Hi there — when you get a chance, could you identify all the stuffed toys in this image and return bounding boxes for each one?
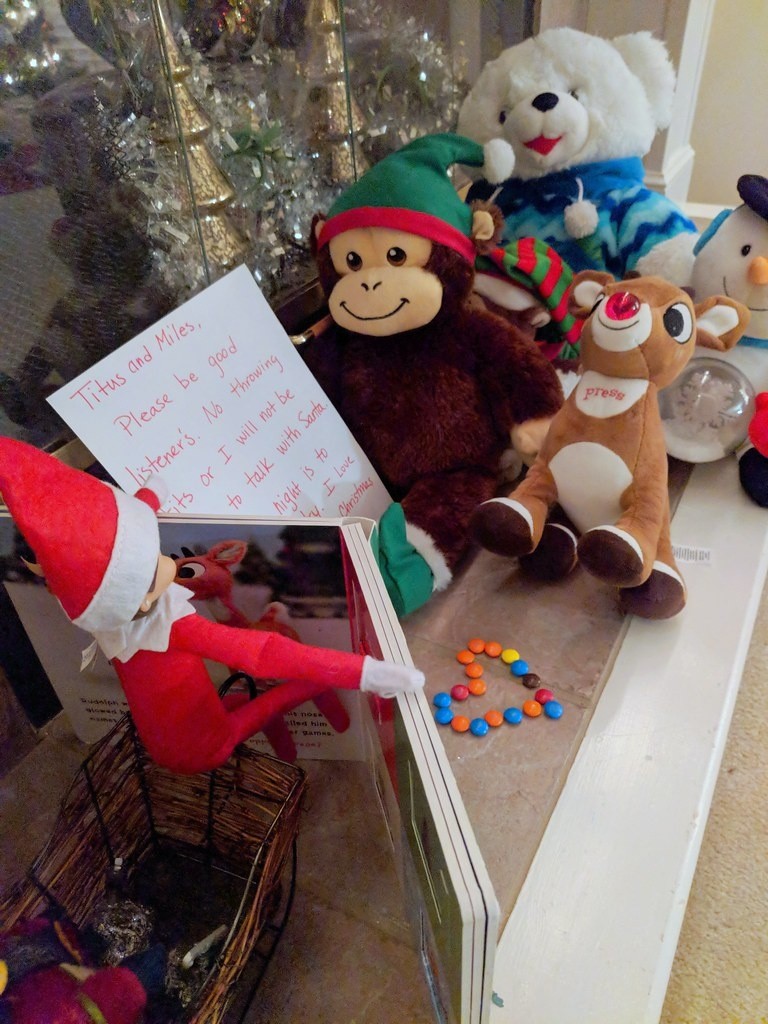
[691,174,768,510]
[296,131,566,622]
[470,270,751,623]
[455,27,700,286]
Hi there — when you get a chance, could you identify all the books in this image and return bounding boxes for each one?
[0,505,501,1024]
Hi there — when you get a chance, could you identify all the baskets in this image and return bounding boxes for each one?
[0,711,308,1024]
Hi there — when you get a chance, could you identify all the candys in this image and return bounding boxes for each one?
[433,639,566,736]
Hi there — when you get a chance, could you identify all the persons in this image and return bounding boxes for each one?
[0,435,424,778]
